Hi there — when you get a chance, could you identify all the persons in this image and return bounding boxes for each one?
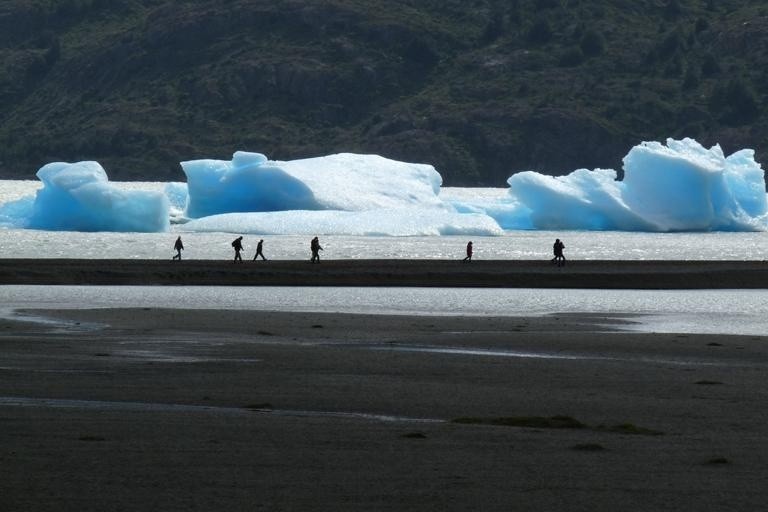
[463,242,472,263]
[232,237,244,260]
[552,239,565,266]
[254,240,267,260]
[311,237,323,264]
[173,237,184,261]
